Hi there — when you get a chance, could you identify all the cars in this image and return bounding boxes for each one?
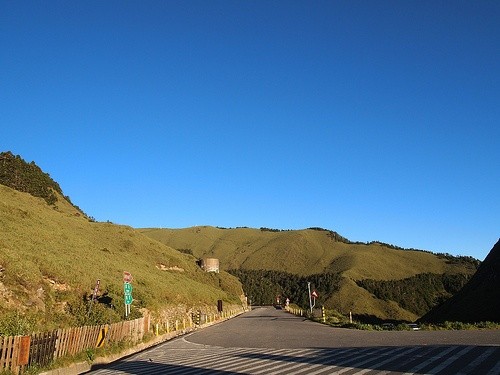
[381,323,395,331]
[406,324,421,331]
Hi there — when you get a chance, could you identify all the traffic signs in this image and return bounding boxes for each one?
[124,272,133,304]
